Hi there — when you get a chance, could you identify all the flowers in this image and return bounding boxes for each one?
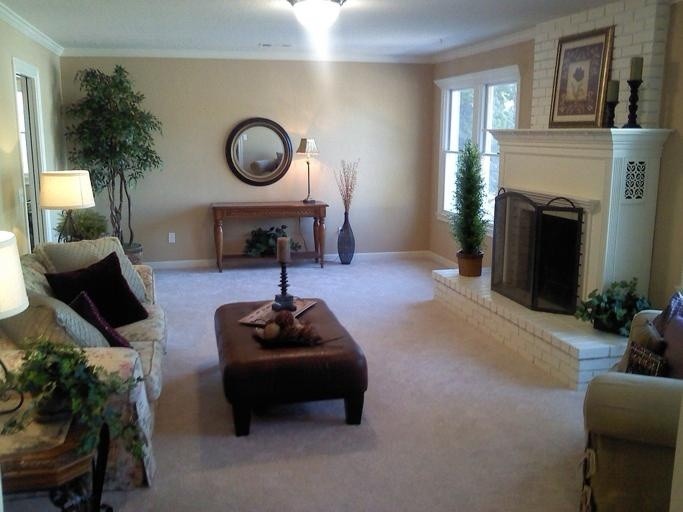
[332,158,361,213]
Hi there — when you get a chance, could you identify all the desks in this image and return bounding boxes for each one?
[211,201,329,273]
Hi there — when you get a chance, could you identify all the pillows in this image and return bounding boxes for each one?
[44,236,147,306]
[625,341,668,376]
[653,292,683,337]
[65,291,134,347]
[44,250,148,328]
[4,289,110,348]
[633,319,665,352]
[661,312,683,380]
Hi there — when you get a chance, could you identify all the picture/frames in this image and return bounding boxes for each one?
[547,26,615,129]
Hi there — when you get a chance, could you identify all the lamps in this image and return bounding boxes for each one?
[0,230,31,321]
[287,1,347,26]
[37,170,94,239]
[296,137,319,202]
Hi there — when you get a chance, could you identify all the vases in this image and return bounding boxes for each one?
[338,213,355,265]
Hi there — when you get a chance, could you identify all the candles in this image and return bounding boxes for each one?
[629,56,643,81]
[607,80,619,101]
[277,236,291,263]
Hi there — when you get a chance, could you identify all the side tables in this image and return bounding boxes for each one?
[1,388,101,504]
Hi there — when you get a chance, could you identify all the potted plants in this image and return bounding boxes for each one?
[451,137,488,277]
[574,277,653,336]
[242,224,301,256]
[59,61,163,265]
[3,342,146,470]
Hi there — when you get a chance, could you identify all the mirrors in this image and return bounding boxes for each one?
[226,117,294,186]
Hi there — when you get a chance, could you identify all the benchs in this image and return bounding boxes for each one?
[214,298,368,437]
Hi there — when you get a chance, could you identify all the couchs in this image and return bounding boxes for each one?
[1,252,167,488]
[582,311,683,512]
[250,152,283,176]
